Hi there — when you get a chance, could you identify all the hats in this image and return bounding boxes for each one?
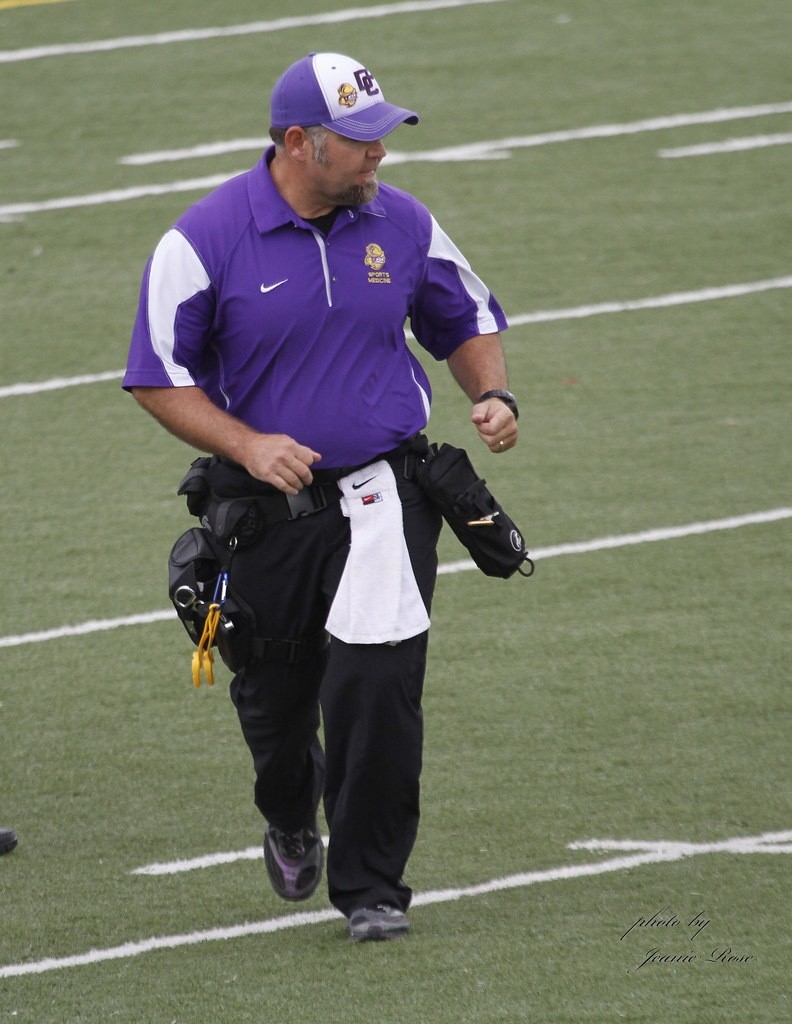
[269,52,418,140]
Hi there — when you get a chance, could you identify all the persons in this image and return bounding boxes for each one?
[121,53,519,941]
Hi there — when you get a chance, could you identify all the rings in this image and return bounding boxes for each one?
[499,440,504,451]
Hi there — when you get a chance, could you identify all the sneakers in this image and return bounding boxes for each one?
[264,826,323,901]
[349,904,407,941]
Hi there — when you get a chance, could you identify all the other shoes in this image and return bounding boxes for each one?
[2,826,17,853]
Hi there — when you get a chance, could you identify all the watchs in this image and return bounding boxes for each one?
[477,389,519,422]
